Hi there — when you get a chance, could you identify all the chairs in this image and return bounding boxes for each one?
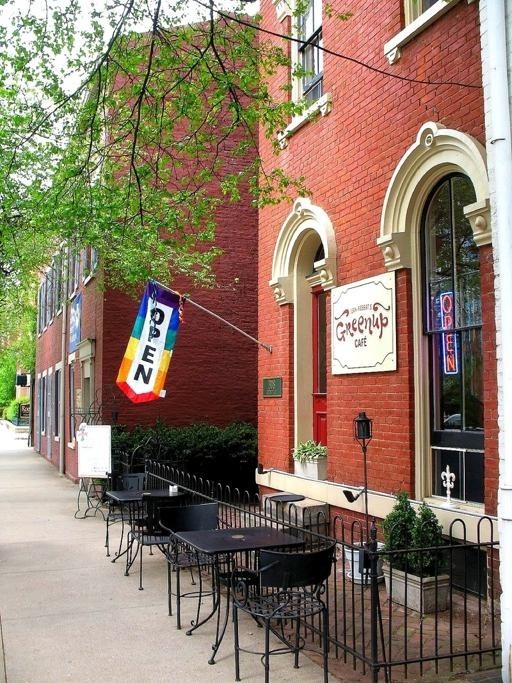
[94,467,153,557]
[157,502,236,631]
[124,493,197,590]
[230,540,334,682]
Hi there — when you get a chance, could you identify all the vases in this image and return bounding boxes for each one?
[344,540,387,579]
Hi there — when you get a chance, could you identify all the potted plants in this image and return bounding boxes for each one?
[379,482,457,616]
[289,439,329,481]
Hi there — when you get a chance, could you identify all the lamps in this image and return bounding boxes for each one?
[258,462,271,474]
[343,487,365,504]
[352,411,374,542]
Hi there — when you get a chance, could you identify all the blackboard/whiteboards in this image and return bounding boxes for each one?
[78,425,112,478]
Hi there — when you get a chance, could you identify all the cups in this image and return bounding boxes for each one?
[169,484,178,492]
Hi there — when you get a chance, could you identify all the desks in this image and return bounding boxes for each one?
[106,488,186,575]
[173,528,307,664]
